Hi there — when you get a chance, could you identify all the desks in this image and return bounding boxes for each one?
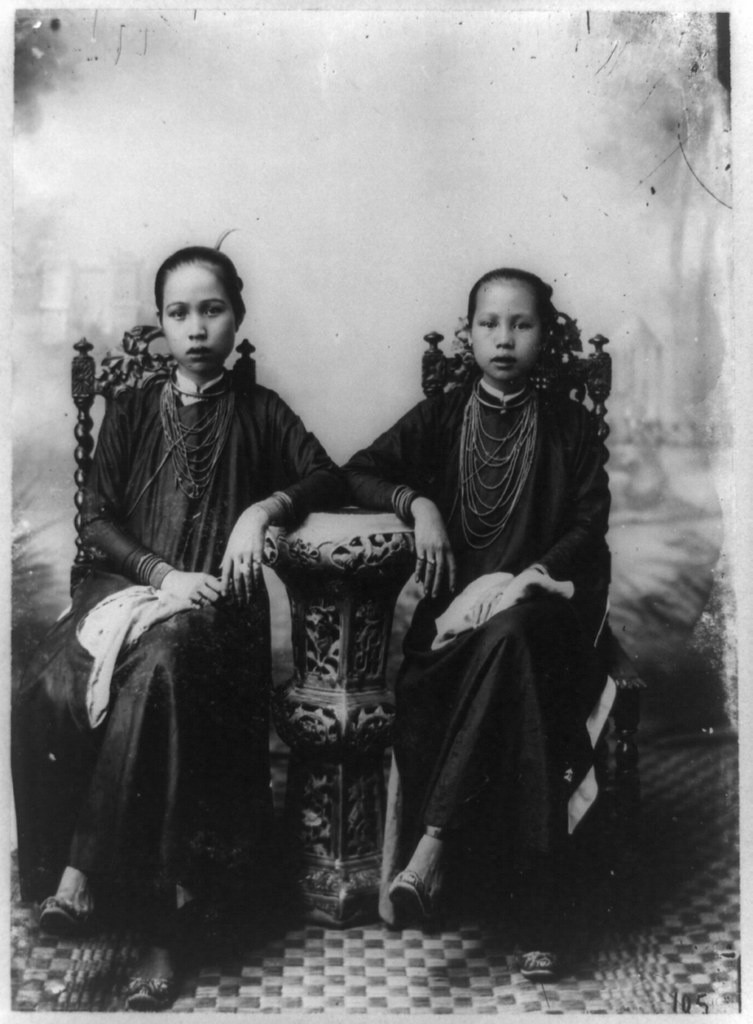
[262,505,418,930]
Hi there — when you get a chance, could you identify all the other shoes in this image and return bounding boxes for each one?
[127,966,177,1006]
[41,895,98,934]
[515,950,563,978]
[388,868,444,919]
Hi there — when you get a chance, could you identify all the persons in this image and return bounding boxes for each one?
[39,247,344,1014]
[338,267,618,975]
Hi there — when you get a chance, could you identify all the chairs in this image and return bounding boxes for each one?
[419,329,650,923]
[69,327,275,911]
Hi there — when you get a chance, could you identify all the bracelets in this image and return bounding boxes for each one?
[391,483,415,523]
[139,554,174,589]
[255,491,294,521]
[533,566,544,574]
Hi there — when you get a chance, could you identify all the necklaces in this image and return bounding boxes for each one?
[458,374,539,549]
[160,362,238,500]
